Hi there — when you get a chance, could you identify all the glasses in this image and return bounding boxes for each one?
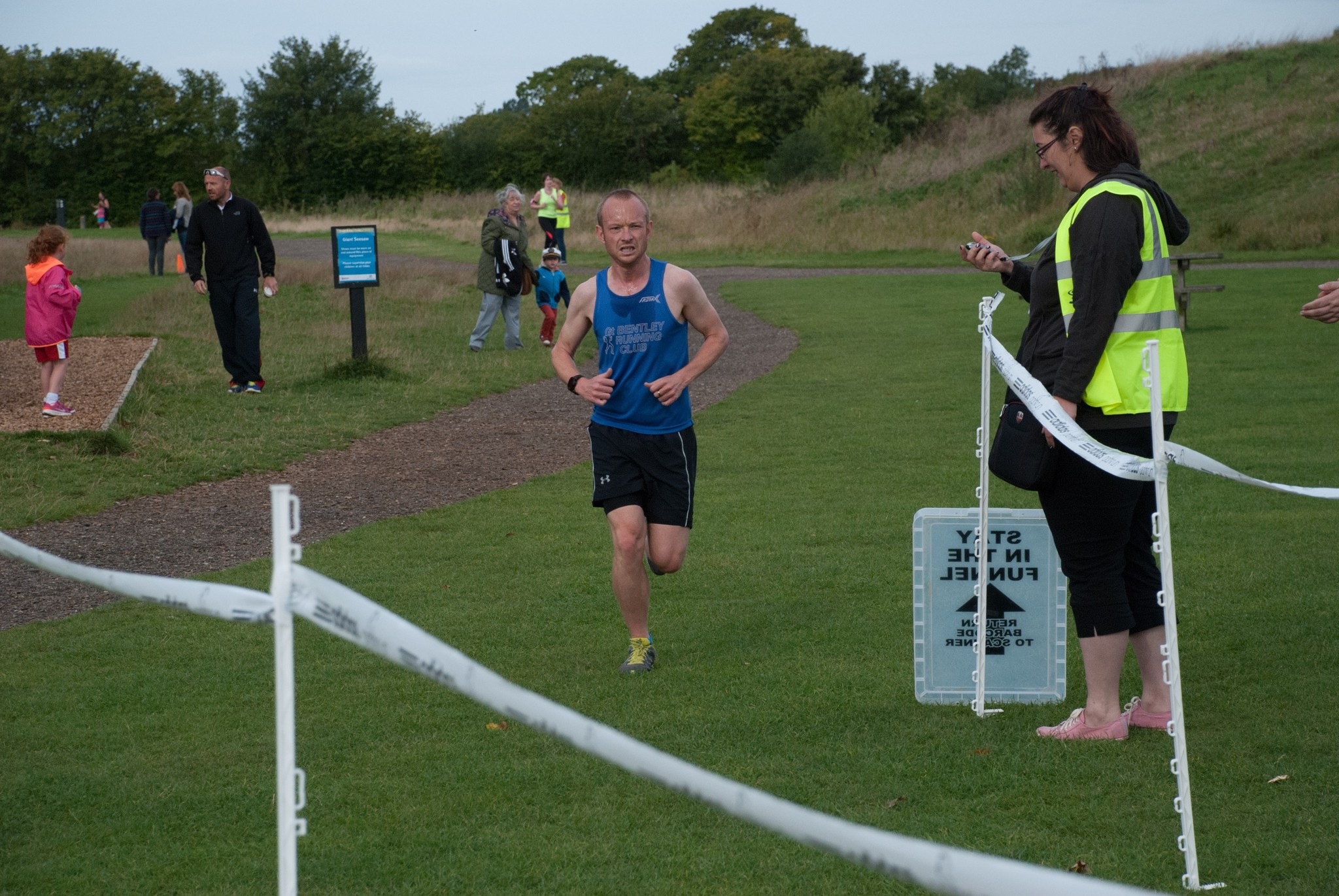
[204,169,228,180]
[1034,132,1067,159]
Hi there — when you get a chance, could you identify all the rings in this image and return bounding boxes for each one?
[657,390,662,397]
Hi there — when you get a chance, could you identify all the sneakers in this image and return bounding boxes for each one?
[644,536,665,575]
[619,634,656,676]
[43,407,76,418]
[42,402,72,416]
[1121,696,1173,731]
[244,381,261,393]
[1037,708,1129,741]
[227,381,246,393]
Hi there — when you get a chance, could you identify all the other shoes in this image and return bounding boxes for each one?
[540,334,550,345]
[558,260,567,266]
[470,346,480,352]
[544,342,556,347]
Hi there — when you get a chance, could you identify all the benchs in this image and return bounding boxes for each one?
[1170,253,1227,331]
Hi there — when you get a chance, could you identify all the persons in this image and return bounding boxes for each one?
[535,247,571,349]
[139,187,171,276]
[171,181,194,272]
[92,192,111,229]
[25,225,81,418]
[469,184,534,352]
[185,167,278,392]
[958,86,1192,741]
[530,173,563,268]
[1300,278,1339,324]
[550,189,729,677]
[551,177,570,266]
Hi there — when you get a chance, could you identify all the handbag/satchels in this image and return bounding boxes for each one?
[989,350,1059,490]
[521,267,532,295]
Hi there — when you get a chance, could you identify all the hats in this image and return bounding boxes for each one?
[542,247,561,263]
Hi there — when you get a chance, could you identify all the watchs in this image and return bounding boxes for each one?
[567,374,585,395]
